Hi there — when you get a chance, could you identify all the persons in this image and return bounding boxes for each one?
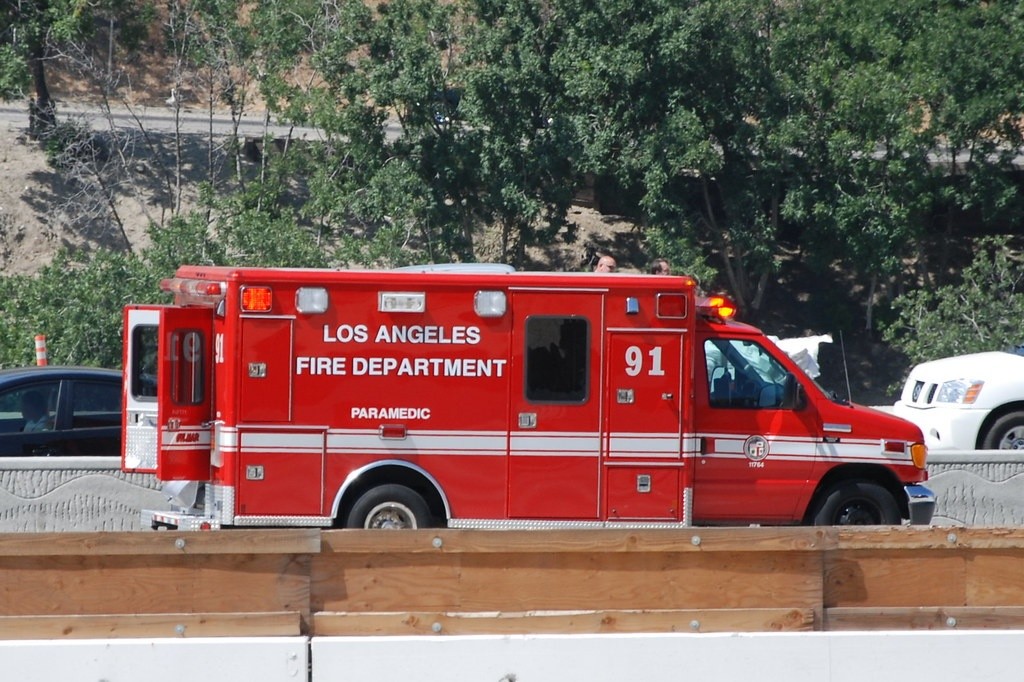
[19,389,52,434]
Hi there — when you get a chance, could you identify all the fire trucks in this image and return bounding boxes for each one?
[119,263,942,534]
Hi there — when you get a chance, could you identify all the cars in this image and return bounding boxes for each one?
[0,366,156,460]
[891,339,1024,450]
